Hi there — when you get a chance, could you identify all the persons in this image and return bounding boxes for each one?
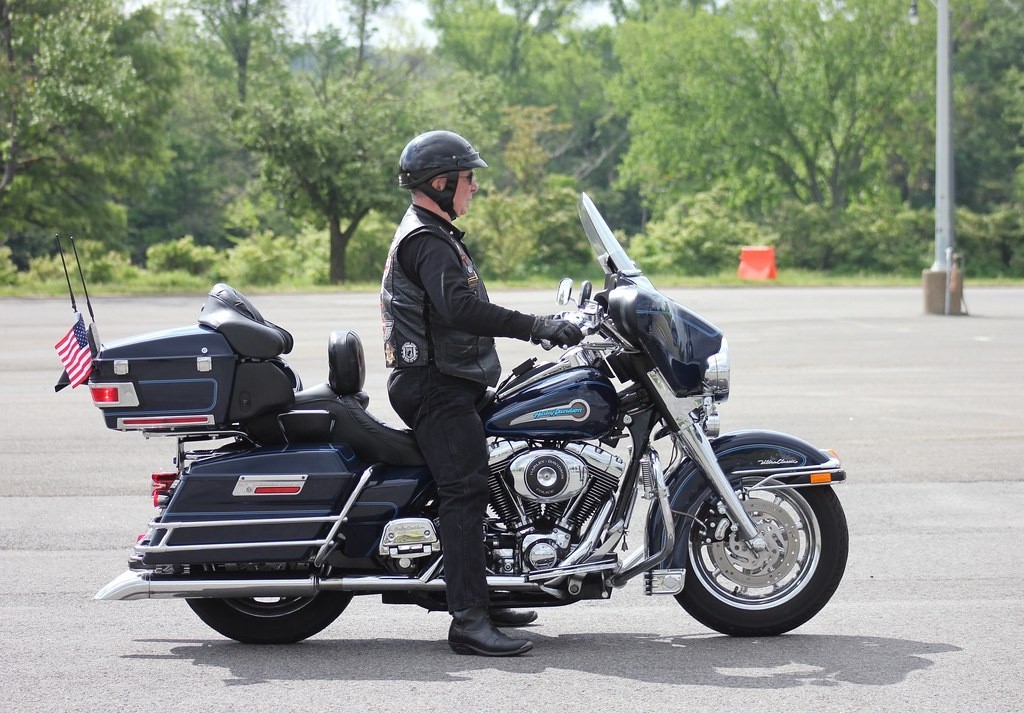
[379,130,585,658]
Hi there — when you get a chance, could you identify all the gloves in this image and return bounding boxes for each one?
[531,316,582,347]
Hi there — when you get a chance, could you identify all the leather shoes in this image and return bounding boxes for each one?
[487,605,537,626]
[448,606,535,656]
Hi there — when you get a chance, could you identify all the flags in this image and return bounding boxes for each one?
[55,316,93,389]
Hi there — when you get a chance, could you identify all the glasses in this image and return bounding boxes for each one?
[433,171,473,186]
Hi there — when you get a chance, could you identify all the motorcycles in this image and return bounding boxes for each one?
[85,189,852,645]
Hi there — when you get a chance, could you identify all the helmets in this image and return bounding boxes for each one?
[398,131,487,190]
